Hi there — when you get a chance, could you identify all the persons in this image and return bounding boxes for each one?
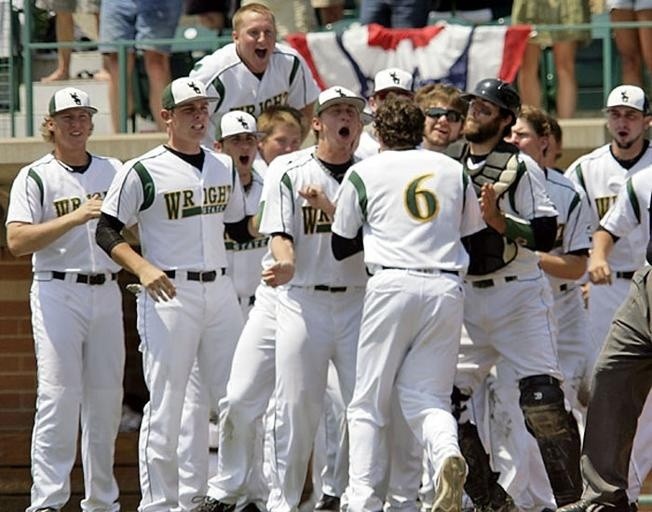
[257,108,304,173]
[258,86,371,512]
[190,121,365,512]
[179,110,288,512]
[355,69,652,512]
[511,1,592,116]
[5,87,140,512]
[31,1,230,134]
[606,0,652,91]
[189,3,320,149]
[94,75,256,512]
[361,1,513,29]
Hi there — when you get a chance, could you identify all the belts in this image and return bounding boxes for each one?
[53,271,116,285]
[164,268,225,282]
[315,284,347,292]
[616,271,634,279]
[463,276,516,287]
[238,296,255,304]
[365,266,459,276]
[560,284,567,290]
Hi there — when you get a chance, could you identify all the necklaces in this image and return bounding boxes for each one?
[314,153,345,179]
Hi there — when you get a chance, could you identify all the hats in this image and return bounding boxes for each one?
[606,84,650,113]
[315,85,365,117]
[460,79,522,125]
[375,68,414,97]
[50,88,97,117]
[159,77,219,110]
[216,109,268,144]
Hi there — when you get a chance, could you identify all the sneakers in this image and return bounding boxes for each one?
[472,486,518,512]
[431,455,467,511]
[126,112,159,133]
[555,497,631,512]
[313,493,340,511]
[189,495,235,511]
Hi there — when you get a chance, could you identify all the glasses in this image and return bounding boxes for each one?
[425,108,466,123]
